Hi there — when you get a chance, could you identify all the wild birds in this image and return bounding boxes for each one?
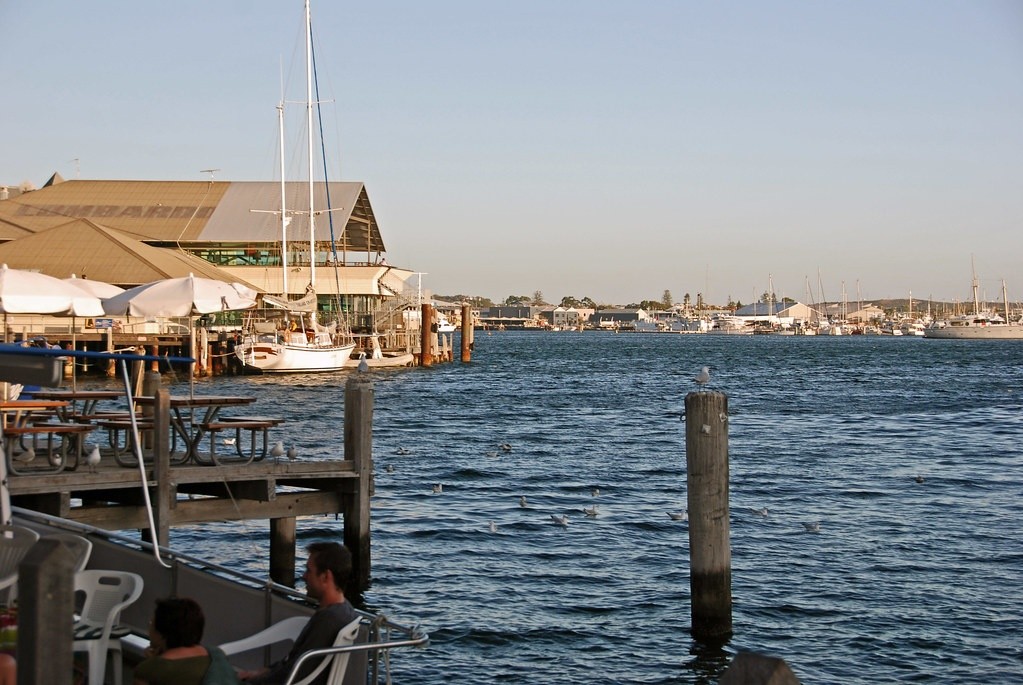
[287,444,297,461]
[222,438,236,445]
[432,483,442,493]
[690,366,709,391]
[479,450,499,457]
[357,354,368,374]
[50,454,62,467]
[386,464,393,473]
[665,509,686,520]
[520,496,528,507]
[488,521,497,532]
[498,444,512,453]
[800,522,821,532]
[397,446,411,455]
[551,514,568,525]
[584,505,601,516]
[269,441,284,465]
[80,443,101,473]
[13,447,35,466]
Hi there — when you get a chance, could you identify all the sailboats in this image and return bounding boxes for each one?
[754,273,881,336]
[235,0,355,372]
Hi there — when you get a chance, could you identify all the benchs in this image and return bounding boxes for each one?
[0,408,285,477]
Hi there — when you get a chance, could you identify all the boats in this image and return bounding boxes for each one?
[629,306,754,335]
[438,319,456,334]
[881,262,1023,339]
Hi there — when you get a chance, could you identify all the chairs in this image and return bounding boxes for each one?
[0,525,363,685]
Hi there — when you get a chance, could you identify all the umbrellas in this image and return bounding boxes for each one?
[0,263,258,466]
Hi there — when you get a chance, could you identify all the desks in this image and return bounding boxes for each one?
[131,394,259,466]
[0,401,71,462]
[19,389,127,457]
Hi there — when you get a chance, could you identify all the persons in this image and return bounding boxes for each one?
[125,594,238,685]
[237,542,353,685]
